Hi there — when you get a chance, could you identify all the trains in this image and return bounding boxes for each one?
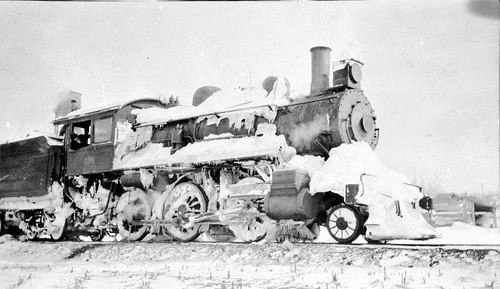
[1,44,438,243]
